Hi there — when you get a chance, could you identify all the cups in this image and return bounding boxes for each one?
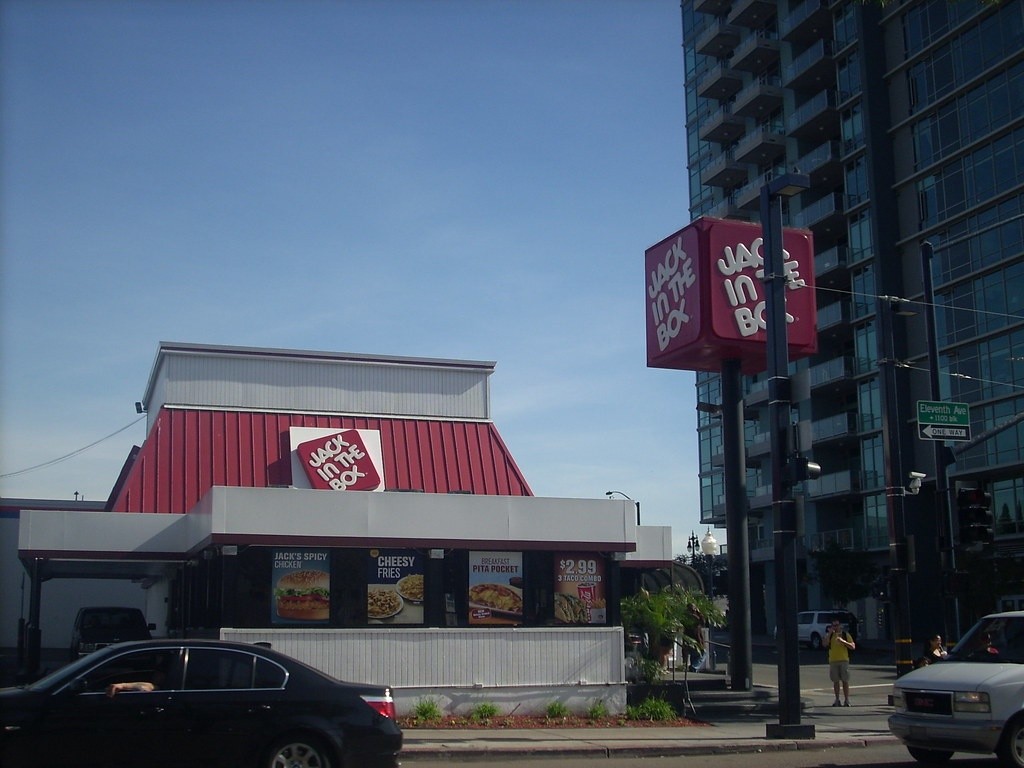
[576,582,596,624]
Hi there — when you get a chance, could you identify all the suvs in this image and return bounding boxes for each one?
[773,608,859,652]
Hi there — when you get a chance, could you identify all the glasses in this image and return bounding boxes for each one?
[833,624,839,626]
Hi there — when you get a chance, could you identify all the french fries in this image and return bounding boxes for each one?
[398,573,423,600]
[590,598,605,609]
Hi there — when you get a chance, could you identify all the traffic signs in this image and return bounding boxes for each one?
[916,399,972,442]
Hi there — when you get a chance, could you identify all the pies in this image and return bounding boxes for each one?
[554,591,588,624]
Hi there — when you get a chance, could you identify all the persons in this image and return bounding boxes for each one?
[823,618,855,707]
[914,633,948,670]
[682,603,707,673]
[982,634,998,653]
[105,652,177,698]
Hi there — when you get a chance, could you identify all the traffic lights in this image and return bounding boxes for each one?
[798,456,823,480]
[957,487,984,542]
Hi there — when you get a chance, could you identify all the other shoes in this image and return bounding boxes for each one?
[844,701,853,707]
[833,699,841,706]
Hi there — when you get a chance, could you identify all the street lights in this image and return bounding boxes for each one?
[877,293,926,681]
[605,491,641,526]
[702,526,717,671]
[687,530,701,569]
[759,172,818,741]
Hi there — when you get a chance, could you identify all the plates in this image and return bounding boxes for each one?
[368,590,404,618]
[396,574,424,602]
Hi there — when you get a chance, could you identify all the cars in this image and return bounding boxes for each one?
[886,610,1024,768]
[67,606,158,662]
[0,637,403,767]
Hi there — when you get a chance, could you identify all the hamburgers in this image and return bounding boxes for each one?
[274,570,330,620]
